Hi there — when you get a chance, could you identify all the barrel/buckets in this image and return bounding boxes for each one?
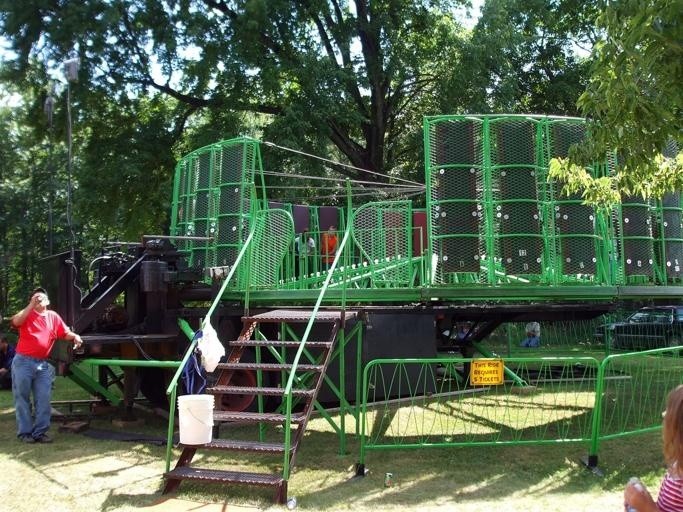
[177,393,215,445]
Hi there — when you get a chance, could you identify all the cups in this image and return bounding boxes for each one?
[34,296,43,306]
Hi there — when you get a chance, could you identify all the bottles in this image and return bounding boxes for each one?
[288,496,297,511]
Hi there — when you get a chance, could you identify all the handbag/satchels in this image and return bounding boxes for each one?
[198,323,226,372]
[181,354,206,394]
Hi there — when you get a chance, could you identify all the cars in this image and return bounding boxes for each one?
[593,306,683,355]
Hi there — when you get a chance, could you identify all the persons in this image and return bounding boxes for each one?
[0,337,16,390]
[520,322,540,347]
[623,385,683,512]
[11,287,83,441]
[295,229,315,280]
[319,226,338,271]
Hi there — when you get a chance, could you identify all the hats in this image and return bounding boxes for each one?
[29,288,47,303]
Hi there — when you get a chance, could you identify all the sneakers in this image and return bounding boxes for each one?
[19,433,53,443]
